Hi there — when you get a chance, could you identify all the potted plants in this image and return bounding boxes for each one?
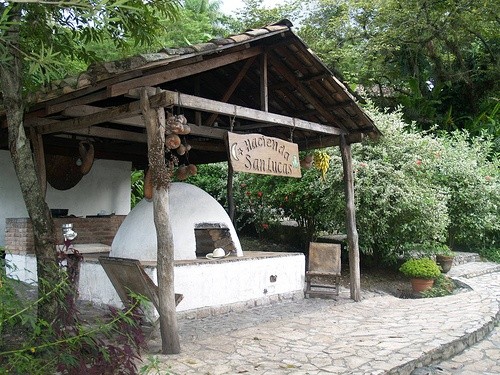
[400,258,441,294]
[439,246,452,272]
[435,246,443,264]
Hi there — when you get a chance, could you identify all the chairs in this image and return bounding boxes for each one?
[305,242,340,299]
[98,256,184,342]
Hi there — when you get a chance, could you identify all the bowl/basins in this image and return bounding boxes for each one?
[213,247,225,257]
